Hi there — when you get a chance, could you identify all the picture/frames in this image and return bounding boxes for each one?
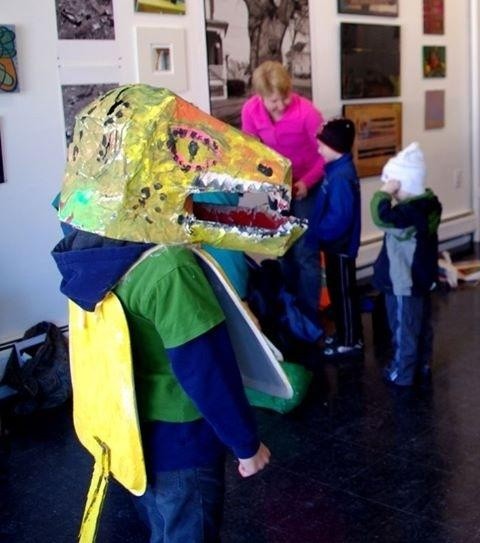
[132,24,189,95]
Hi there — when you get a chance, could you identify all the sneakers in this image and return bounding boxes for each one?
[322,332,366,360]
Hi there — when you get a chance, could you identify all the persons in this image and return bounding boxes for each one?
[243,61,332,348]
[369,141,443,388]
[311,114,366,355]
[48,83,310,540]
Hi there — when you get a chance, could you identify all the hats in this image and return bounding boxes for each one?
[312,117,356,157]
[379,142,429,200]
